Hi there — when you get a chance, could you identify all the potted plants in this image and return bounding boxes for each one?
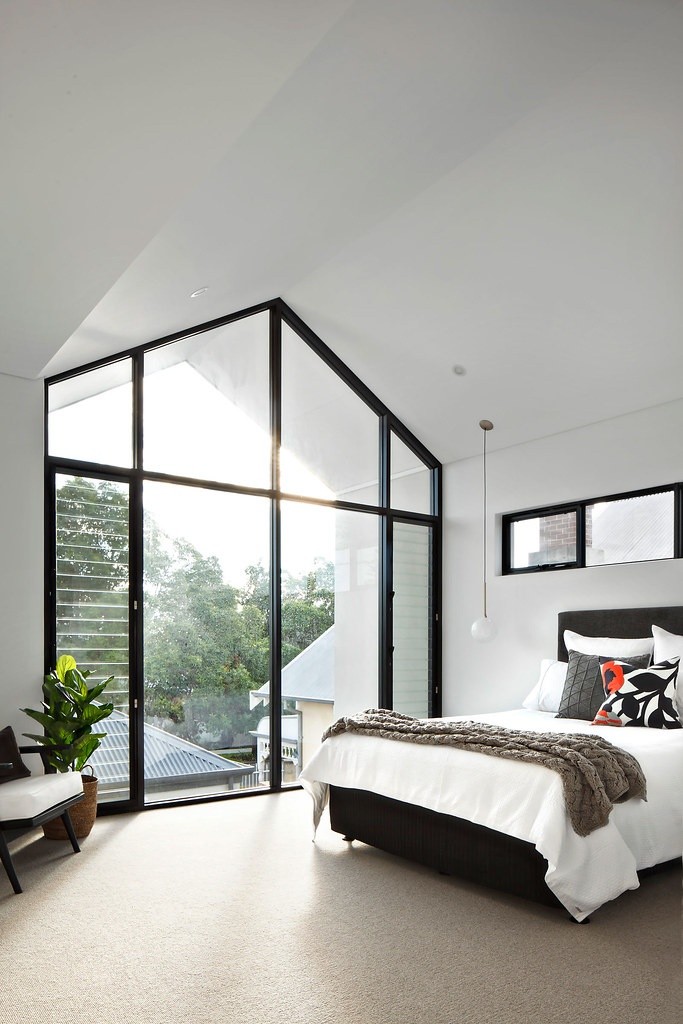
[19,655,115,840]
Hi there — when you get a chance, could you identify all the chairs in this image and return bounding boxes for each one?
[0,726,85,894]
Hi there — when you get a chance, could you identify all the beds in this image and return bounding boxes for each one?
[299,605,683,925]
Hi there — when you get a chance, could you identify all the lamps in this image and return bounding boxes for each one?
[472,419,497,642]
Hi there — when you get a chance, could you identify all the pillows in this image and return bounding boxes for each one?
[521,659,569,711]
[563,630,654,667]
[652,624,683,726]
[591,655,682,729]
[554,648,651,721]
